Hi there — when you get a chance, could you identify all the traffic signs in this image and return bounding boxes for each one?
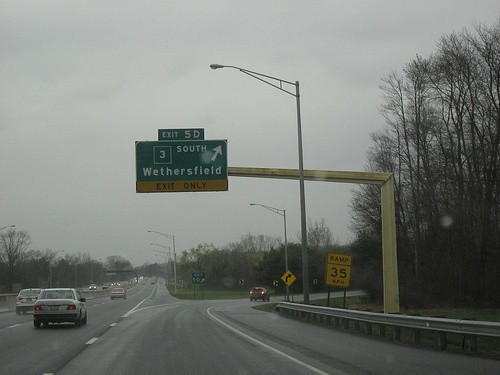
[135,139,228,195]
[191,273,206,285]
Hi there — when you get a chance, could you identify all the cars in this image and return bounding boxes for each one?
[110,288,127,300]
[15,288,48,316]
[89,284,96,290]
[33,288,87,328]
[102,284,109,289]
[249,287,270,302]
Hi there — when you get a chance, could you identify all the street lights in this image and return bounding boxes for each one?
[250,203,289,302]
[148,231,176,295]
[49,250,65,288]
[209,64,309,305]
[150,244,172,288]
